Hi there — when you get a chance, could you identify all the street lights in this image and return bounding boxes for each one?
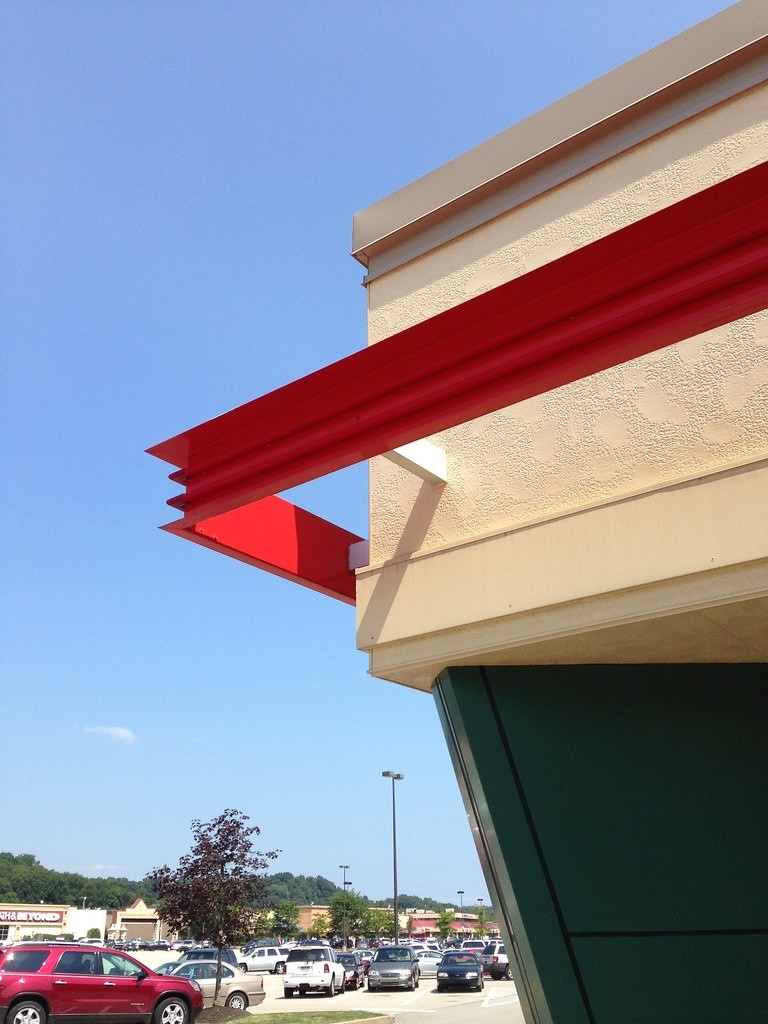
[383,771,405,945]
[478,899,483,908]
[458,891,464,911]
[339,866,352,948]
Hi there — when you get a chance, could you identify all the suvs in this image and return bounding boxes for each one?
[482,944,514,981]
[238,947,289,974]
[179,948,238,967]
[284,945,346,998]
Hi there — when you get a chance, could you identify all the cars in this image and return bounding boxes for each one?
[243,938,503,953]
[79,938,215,951]
[0,941,204,1024]
[368,946,419,991]
[416,949,444,977]
[437,952,485,991]
[337,952,365,989]
[152,959,266,1011]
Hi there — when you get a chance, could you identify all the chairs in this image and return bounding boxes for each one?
[192,968,199,978]
